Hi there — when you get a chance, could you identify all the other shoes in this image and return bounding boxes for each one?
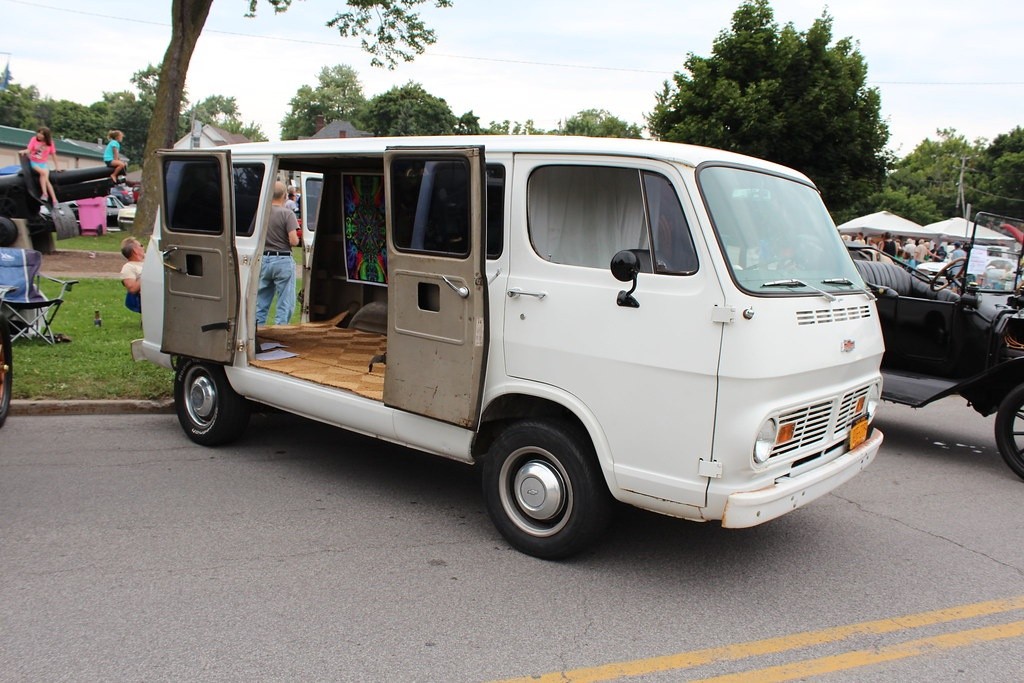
[53,200,61,210]
[40,194,48,201]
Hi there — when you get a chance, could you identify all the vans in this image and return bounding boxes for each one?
[123,131,887,562]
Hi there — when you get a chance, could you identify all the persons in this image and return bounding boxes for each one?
[18,126,65,210]
[117,236,145,294]
[103,130,127,182]
[842,232,966,296]
[254,179,301,324]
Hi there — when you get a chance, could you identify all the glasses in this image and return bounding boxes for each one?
[140,244,143,246]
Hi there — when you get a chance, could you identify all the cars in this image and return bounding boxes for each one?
[39,180,140,231]
[841,210,1024,484]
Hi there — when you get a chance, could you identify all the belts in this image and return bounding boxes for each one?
[263,250,291,256]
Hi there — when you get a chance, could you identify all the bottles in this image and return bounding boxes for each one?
[94,310,101,327]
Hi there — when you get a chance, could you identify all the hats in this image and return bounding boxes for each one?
[952,242,961,246]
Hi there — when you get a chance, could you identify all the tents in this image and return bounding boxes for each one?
[925,217,1016,271]
[838,210,946,240]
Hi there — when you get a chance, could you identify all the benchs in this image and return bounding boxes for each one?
[852,259,959,302]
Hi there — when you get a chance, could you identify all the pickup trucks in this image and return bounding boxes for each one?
[912,245,1021,281]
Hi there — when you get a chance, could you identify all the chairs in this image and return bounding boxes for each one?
[0,246,80,346]
